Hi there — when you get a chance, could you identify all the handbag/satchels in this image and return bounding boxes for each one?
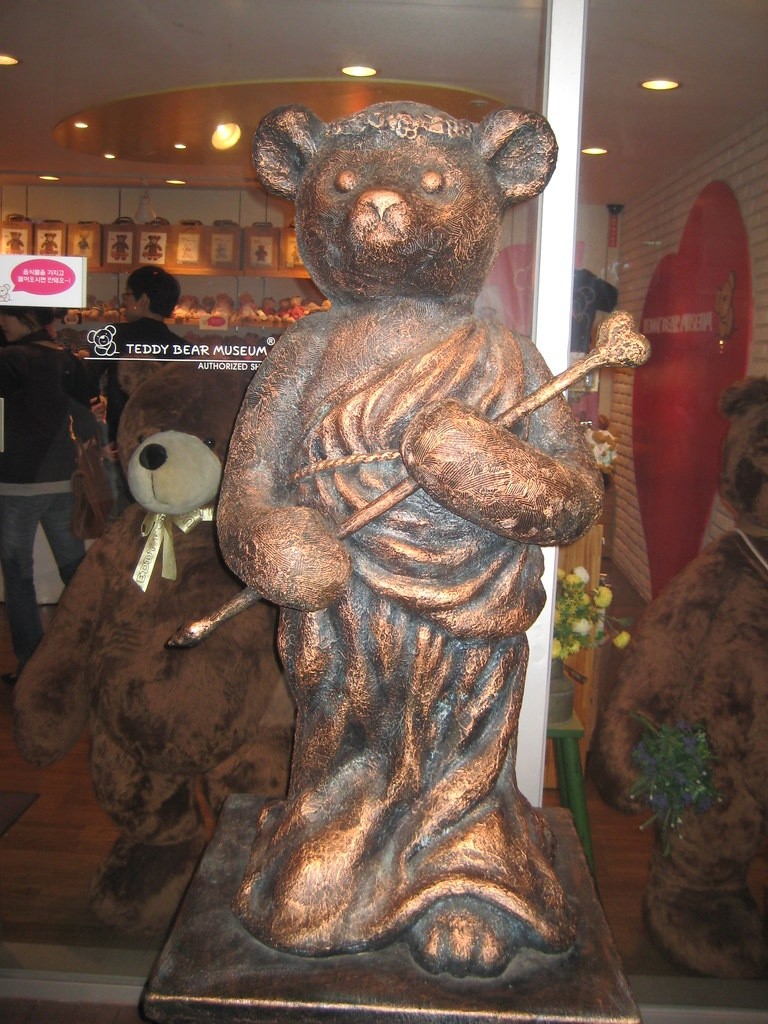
[70,435,115,540]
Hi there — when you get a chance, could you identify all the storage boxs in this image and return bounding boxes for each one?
[0,214,308,272]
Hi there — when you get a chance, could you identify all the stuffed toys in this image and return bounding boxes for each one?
[8,347,304,948]
[57,293,130,425]
[164,292,331,364]
[212,97,602,986]
[586,376,767,983]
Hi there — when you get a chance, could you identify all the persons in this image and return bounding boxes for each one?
[85,266,192,462]
[0,307,99,685]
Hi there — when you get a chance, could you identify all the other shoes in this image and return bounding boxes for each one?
[0,672,19,686]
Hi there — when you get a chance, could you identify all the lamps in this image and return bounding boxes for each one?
[136,180,157,224]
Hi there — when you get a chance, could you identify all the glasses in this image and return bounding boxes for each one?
[121,293,135,302]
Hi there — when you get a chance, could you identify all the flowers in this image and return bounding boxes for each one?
[552,565,633,660]
[627,711,729,853]
